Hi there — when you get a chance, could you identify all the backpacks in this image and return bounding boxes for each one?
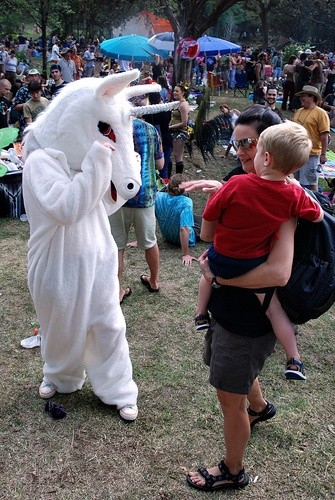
[276,208,335,325]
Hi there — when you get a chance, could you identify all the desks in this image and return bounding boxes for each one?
[0,170,26,219]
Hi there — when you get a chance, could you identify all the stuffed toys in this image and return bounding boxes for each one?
[19,68,162,422]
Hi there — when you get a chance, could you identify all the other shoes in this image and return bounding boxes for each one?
[39,380,54,398]
[119,404,138,421]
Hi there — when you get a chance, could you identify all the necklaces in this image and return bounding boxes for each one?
[298,106,316,125]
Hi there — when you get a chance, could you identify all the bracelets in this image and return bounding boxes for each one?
[321,154,327,156]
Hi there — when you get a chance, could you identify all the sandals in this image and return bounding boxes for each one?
[247,401,276,427]
[187,460,249,491]
[195,314,210,331]
[285,358,306,381]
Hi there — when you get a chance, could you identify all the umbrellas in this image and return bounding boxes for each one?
[100,32,163,62]
[147,31,182,51]
[195,34,244,70]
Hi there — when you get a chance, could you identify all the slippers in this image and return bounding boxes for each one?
[120,287,131,304]
[141,275,160,293]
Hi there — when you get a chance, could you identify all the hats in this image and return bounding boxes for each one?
[60,48,73,54]
[29,69,40,75]
[294,86,321,103]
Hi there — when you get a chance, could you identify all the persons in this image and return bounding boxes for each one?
[291,84,331,191]
[166,83,188,172]
[116,52,173,103]
[195,44,270,106]
[267,86,284,122]
[0,65,68,138]
[0,31,119,83]
[110,96,159,306]
[270,50,335,127]
[181,102,281,491]
[219,104,241,127]
[195,122,323,380]
[154,174,206,268]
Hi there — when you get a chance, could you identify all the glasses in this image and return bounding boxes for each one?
[231,138,258,151]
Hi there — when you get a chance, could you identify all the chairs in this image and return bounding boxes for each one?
[232,73,250,98]
[213,76,225,96]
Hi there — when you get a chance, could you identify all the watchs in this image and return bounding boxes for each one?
[211,274,221,289]
